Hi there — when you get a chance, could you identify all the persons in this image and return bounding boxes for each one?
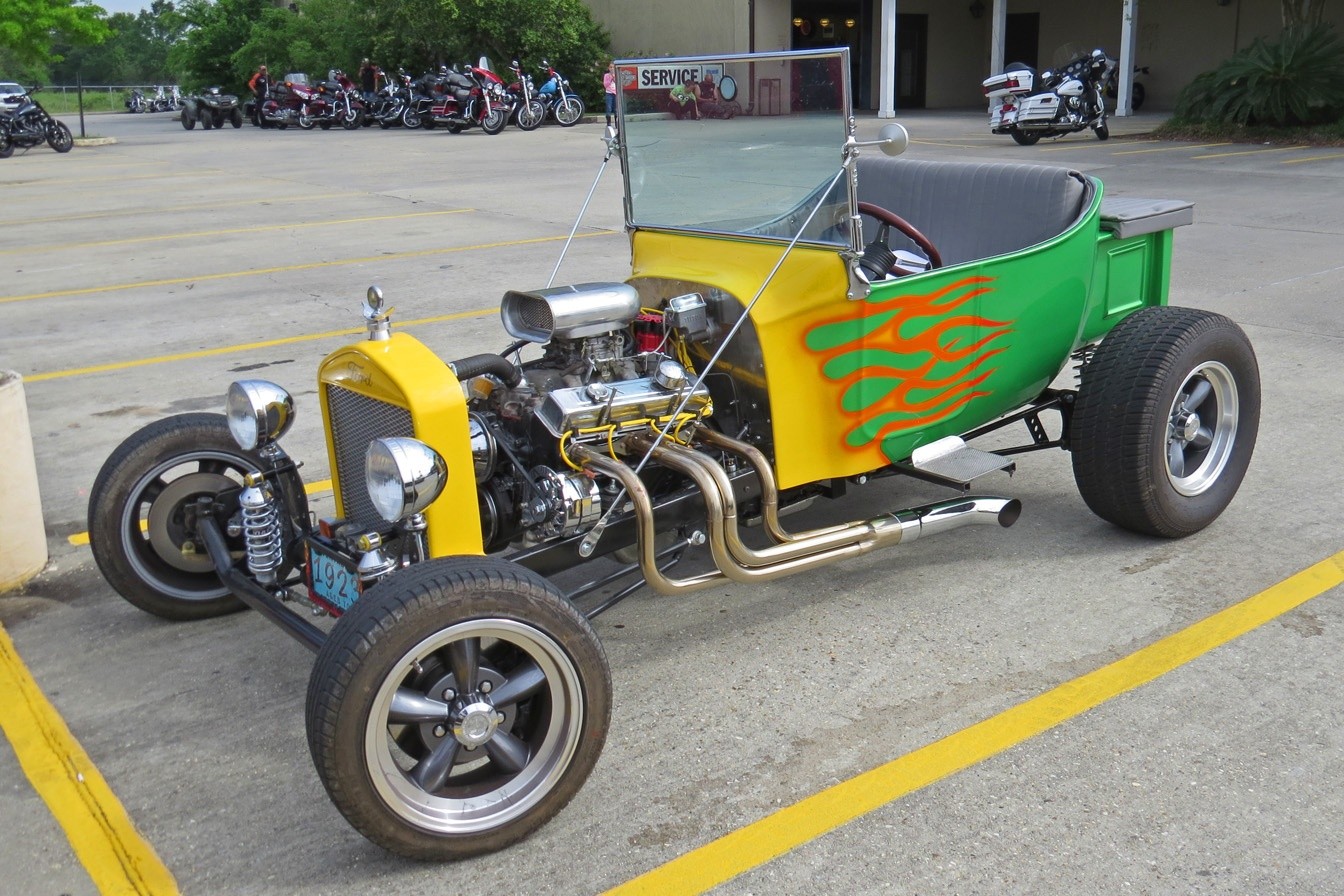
[666,79,700,121]
[357,58,386,128]
[694,73,735,120]
[603,62,619,129]
[248,65,275,129]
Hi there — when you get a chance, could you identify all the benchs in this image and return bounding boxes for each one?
[829,154,1087,278]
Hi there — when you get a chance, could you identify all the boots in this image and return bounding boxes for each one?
[615,116,619,128]
[606,116,611,126]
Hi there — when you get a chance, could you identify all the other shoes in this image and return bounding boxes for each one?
[671,102,733,122]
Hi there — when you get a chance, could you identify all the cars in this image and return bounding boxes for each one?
[0,82,31,118]
[86,49,1260,867]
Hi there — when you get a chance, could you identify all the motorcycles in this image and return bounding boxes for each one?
[125,58,589,135]
[1089,49,1150,113]
[981,49,1119,147]
[0,84,73,159]
